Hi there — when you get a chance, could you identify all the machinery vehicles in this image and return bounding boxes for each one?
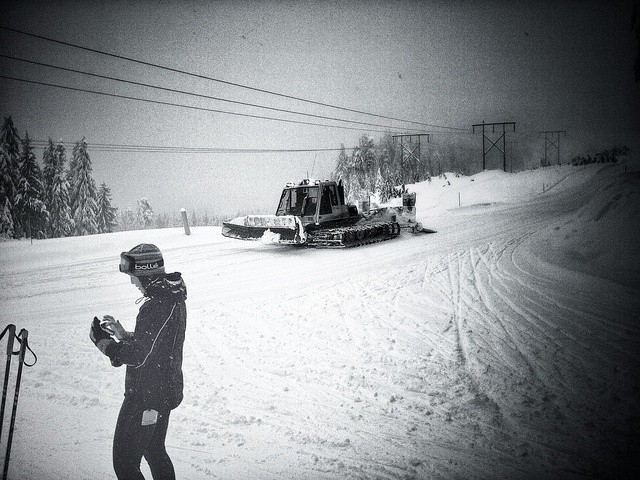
[220,177,437,248]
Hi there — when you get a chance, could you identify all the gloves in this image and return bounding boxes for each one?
[101,315,126,340]
[90,317,114,355]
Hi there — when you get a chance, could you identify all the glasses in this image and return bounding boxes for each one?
[119,252,164,271]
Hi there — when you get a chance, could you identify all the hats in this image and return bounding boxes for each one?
[129,244,164,276]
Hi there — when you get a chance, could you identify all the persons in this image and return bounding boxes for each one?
[89,244,188,476]
